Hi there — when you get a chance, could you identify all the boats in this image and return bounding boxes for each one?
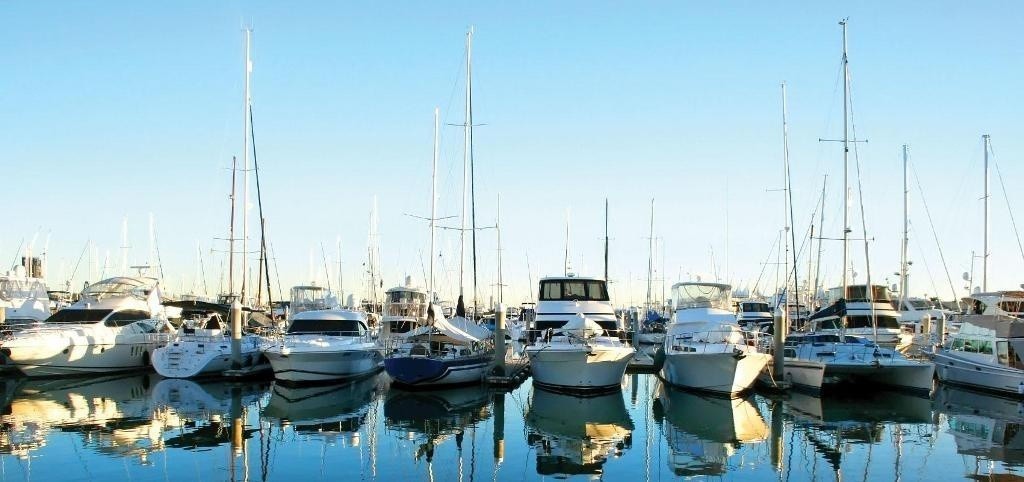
[0,375,1023,481]
[919,291,1024,400]
[524,313,636,393]
[652,283,776,398]
[255,309,385,387]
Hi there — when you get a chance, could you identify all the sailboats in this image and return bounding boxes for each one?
[760,16,936,400]
[1,28,1024,379]
[382,23,516,384]
